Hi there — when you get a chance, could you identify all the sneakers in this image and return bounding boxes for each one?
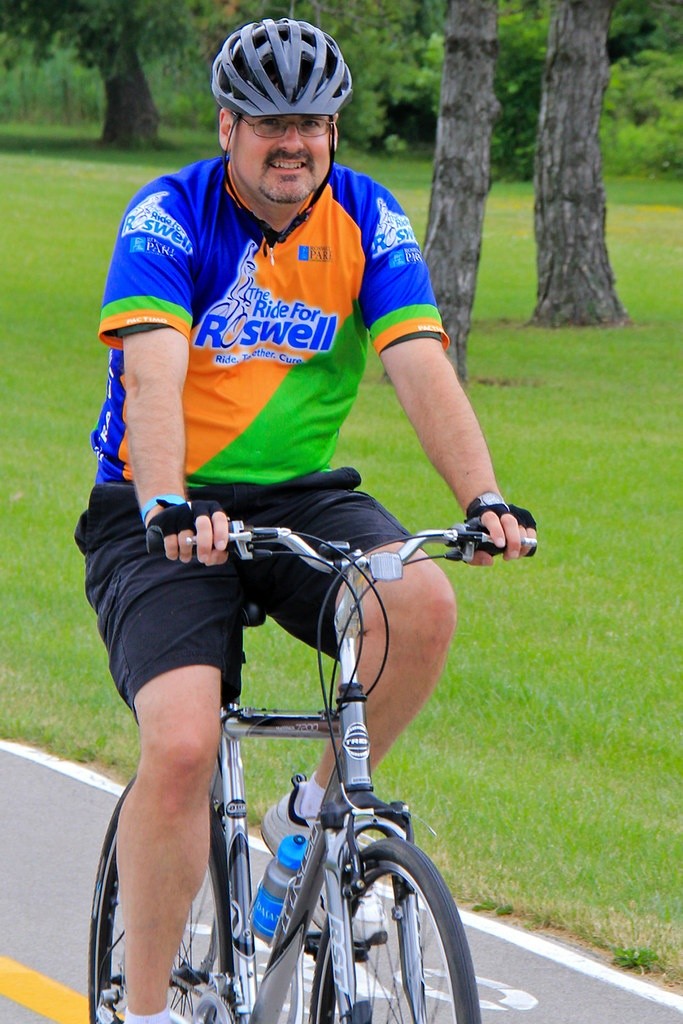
[260,774,389,943]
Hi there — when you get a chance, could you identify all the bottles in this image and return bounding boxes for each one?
[247,833,309,946]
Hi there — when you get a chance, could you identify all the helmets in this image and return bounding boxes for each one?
[210,18,354,117]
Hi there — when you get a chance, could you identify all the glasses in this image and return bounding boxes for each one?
[229,110,333,138]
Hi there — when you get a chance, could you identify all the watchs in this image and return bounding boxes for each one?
[466,490,507,521]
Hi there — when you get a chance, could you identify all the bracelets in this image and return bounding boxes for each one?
[140,495,189,526]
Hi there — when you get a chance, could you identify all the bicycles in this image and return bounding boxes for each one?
[86,521,535,1024]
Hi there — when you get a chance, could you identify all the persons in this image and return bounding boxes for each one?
[73,17,542,1021]
